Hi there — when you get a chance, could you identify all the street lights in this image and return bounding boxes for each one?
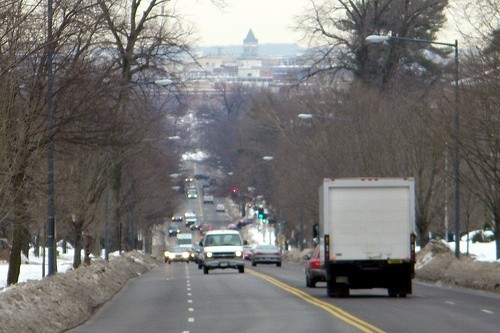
[228,171,246,217]
[298,113,358,178]
[109,79,172,250]
[262,156,304,251]
[365,34,460,259]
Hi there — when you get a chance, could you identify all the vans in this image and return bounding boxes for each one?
[198,229,248,274]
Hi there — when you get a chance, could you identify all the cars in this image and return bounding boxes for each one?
[303,243,326,288]
[216,203,225,212]
[163,211,213,265]
[250,243,283,267]
[183,177,215,204]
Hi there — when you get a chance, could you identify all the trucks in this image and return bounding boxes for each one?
[313,177,417,298]
